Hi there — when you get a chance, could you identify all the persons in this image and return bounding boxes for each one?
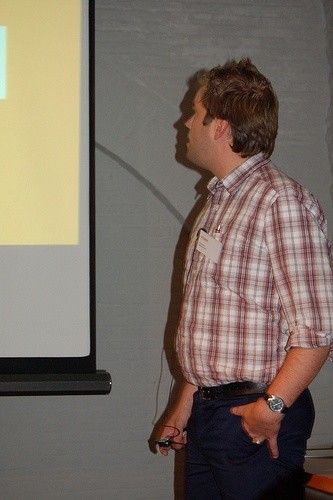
[153,55,333,500]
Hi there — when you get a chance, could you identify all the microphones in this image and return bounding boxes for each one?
[197,227,207,237]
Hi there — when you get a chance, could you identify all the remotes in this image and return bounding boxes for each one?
[162,426,187,447]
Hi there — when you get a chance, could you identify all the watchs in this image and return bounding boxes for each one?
[262,392,290,414]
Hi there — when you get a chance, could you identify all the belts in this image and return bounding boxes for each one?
[199,381,270,400]
